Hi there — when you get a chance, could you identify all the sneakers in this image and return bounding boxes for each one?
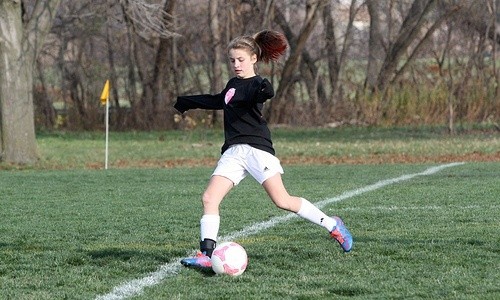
[180,250,216,278]
[329,216,353,252]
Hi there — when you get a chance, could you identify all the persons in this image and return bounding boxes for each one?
[174,30,352,277]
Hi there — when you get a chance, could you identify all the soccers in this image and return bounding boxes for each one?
[211,242,248,276]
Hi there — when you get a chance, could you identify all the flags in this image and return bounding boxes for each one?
[101,79,108,104]
